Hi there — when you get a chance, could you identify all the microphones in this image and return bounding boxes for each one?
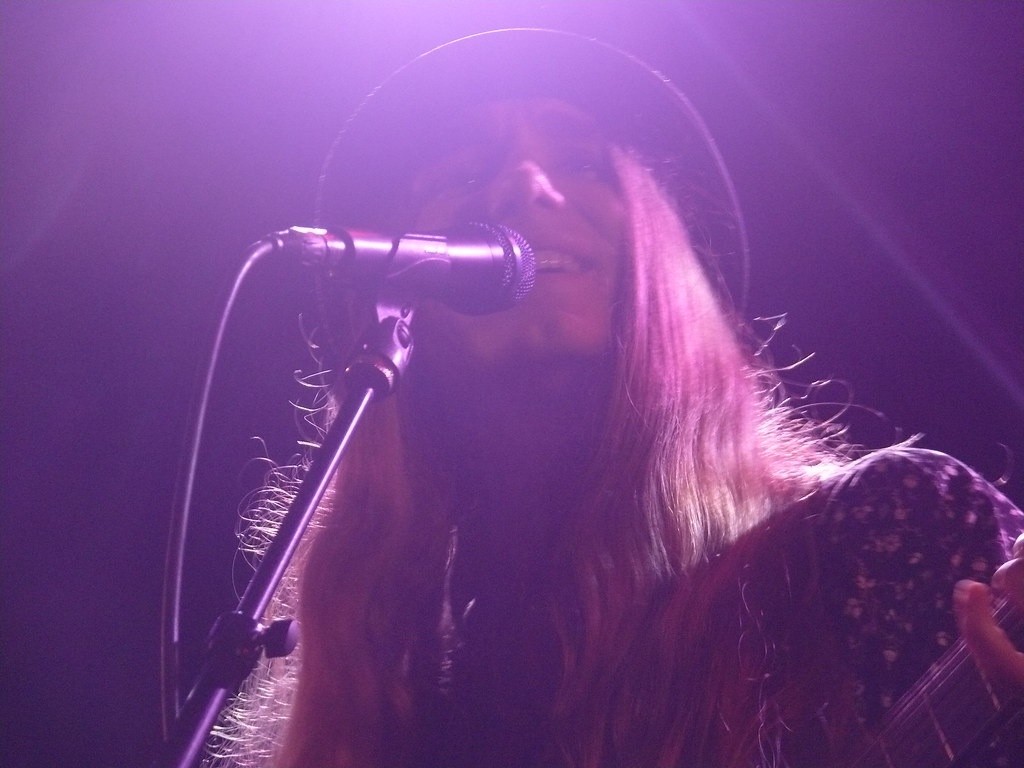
[273,219,536,315]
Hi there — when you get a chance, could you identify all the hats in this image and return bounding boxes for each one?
[312,27,747,343]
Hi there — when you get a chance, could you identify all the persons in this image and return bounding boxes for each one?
[203,24,1024,768]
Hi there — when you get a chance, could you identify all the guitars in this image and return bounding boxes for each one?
[838,592,1024,768]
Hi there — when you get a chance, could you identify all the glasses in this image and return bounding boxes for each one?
[407,522,484,704]
